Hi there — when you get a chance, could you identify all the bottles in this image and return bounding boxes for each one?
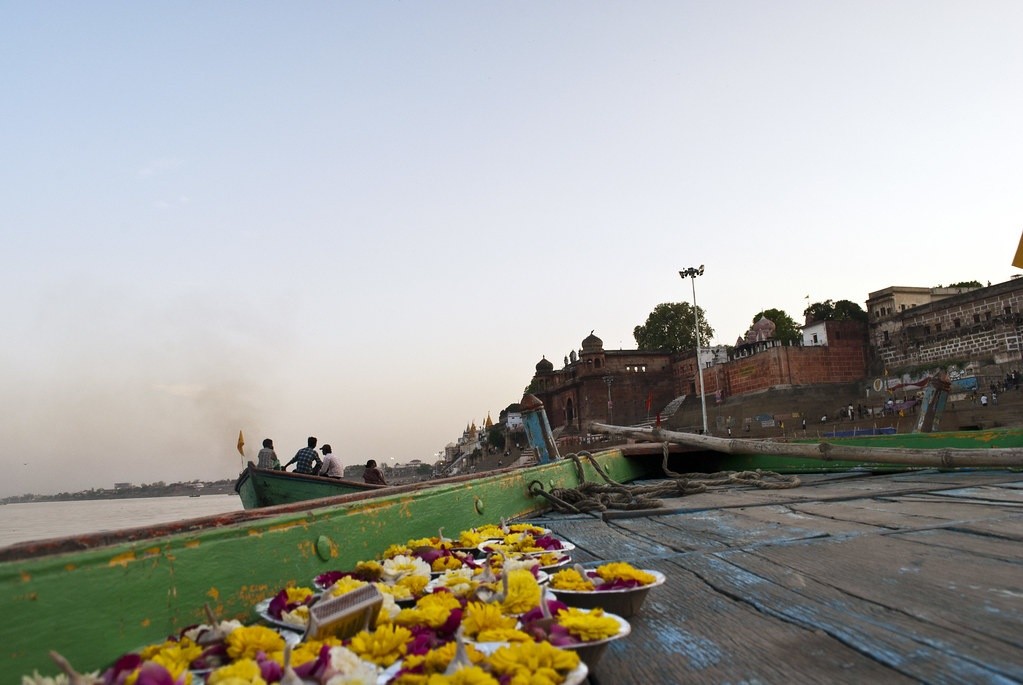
[273,459,281,470]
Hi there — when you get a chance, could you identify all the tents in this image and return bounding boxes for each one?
[888,377,928,402]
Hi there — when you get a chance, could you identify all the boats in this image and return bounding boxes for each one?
[0,432,1022,685]
[235,461,391,511]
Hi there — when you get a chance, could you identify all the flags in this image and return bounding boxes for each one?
[237,430,246,456]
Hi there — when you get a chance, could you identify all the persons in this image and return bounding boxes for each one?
[727,427,732,437]
[839,393,924,423]
[412,443,520,479]
[777,421,784,438]
[281,437,323,475]
[363,460,387,485]
[970,369,1023,407]
[319,444,344,479]
[746,424,750,433]
[258,439,280,470]
[821,415,827,423]
[801,419,806,429]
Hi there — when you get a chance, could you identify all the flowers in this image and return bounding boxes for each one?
[23,522,657,685]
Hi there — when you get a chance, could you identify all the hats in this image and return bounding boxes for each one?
[320,444,331,450]
